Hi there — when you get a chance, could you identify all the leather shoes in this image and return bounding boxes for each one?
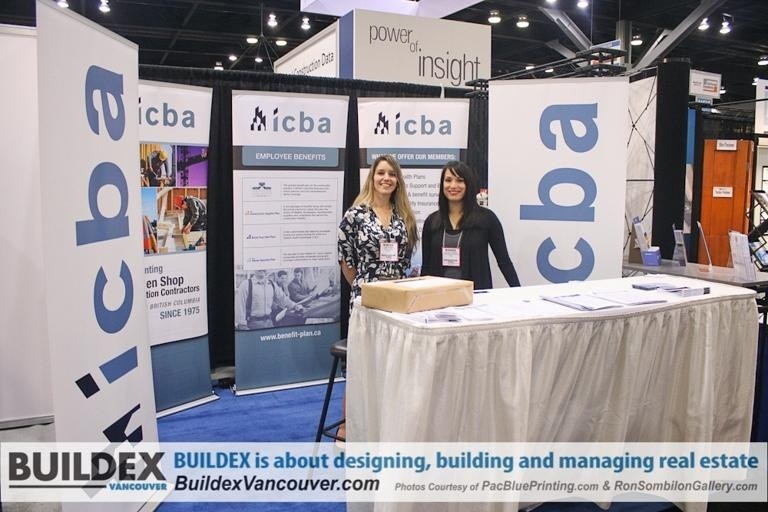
[335,428,345,442]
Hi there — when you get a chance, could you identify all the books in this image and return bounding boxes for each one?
[592,289,668,308]
[543,291,622,311]
[632,280,671,290]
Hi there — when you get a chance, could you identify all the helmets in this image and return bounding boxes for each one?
[159,151,168,161]
[176,195,184,209]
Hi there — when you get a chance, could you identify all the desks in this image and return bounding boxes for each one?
[345,274,759,512]
[622,256,768,287]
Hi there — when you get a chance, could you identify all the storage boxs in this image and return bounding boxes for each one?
[362,275,474,313]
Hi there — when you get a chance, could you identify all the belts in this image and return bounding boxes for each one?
[248,315,272,321]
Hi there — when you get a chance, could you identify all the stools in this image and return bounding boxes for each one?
[307,338,347,484]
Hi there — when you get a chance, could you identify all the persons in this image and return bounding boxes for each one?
[275,270,290,297]
[148,150,171,186]
[337,156,421,452]
[423,161,522,288]
[235,271,306,329]
[290,268,317,306]
[174,195,208,245]
[138,160,149,188]
